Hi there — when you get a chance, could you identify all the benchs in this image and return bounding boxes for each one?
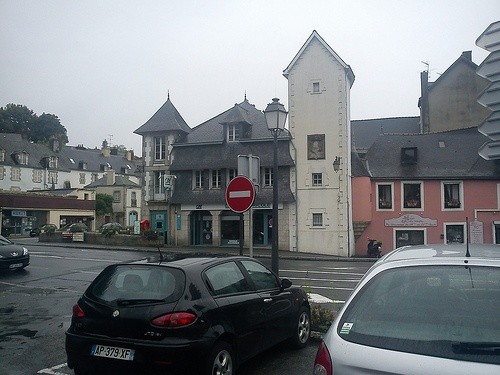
[121,274,163,298]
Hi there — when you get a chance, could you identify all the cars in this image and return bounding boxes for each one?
[100,223,122,231]
[314,244,500,374]
[57,223,89,234]
[65,254,312,375]
[30,224,58,238]
[0,235,30,271]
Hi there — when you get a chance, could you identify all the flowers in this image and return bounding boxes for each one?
[449,198,457,205]
[407,197,420,204]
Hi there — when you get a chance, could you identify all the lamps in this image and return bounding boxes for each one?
[332,156,340,174]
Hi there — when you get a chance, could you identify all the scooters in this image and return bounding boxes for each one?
[367,238,381,258]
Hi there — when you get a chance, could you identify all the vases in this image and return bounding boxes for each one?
[447,201,460,207]
[407,202,418,207]
[381,202,392,206]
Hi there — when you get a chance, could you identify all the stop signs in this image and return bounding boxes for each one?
[226,176,255,213]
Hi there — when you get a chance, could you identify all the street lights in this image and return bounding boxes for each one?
[265,98,289,276]
